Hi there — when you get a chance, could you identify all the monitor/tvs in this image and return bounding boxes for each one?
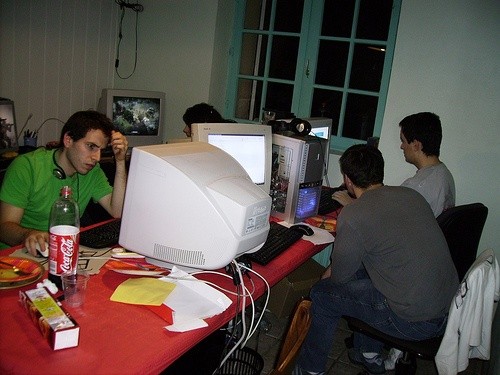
[278,117,332,176]
[99,87,272,270]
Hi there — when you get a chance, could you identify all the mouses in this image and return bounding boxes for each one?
[290,225,314,236]
[35,241,49,257]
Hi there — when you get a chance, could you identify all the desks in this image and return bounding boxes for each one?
[0,205,343,375]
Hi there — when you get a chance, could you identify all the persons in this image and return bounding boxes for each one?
[332,112,456,218]
[283,144,461,375]
[183,103,223,137]
[0,111,128,257]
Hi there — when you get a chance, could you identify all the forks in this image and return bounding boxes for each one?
[0,260,32,276]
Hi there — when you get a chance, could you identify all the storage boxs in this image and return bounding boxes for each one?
[267,257,327,319]
[18,286,80,351]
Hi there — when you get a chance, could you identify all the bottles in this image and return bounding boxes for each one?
[48,186,80,300]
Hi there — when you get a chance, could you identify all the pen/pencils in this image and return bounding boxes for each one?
[55,288,79,303]
[23,129,39,139]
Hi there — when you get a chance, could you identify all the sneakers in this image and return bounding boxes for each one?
[290,365,325,375]
[348,351,384,372]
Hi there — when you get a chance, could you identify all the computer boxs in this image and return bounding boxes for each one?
[269,130,329,223]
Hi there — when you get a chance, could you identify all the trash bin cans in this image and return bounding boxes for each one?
[214,344,264,375]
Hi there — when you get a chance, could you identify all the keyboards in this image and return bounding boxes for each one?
[318,186,342,215]
[244,221,303,266]
[78,218,122,249]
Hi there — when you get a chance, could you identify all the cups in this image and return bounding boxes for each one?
[264,111,275,122]
[23,136,37,147]
[61,270,90,308]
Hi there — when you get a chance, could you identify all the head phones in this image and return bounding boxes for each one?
[53,148,66,180]
[267,118,312,136]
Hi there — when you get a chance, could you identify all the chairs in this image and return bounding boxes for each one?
[342,247,495,375]
[436,202,488,282]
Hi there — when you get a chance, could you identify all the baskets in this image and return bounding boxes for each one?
[215,344,264,375]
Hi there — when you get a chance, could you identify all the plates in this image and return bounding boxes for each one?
[104,259,167,277]
[303,214,337,233]
[0,256,45,288]
